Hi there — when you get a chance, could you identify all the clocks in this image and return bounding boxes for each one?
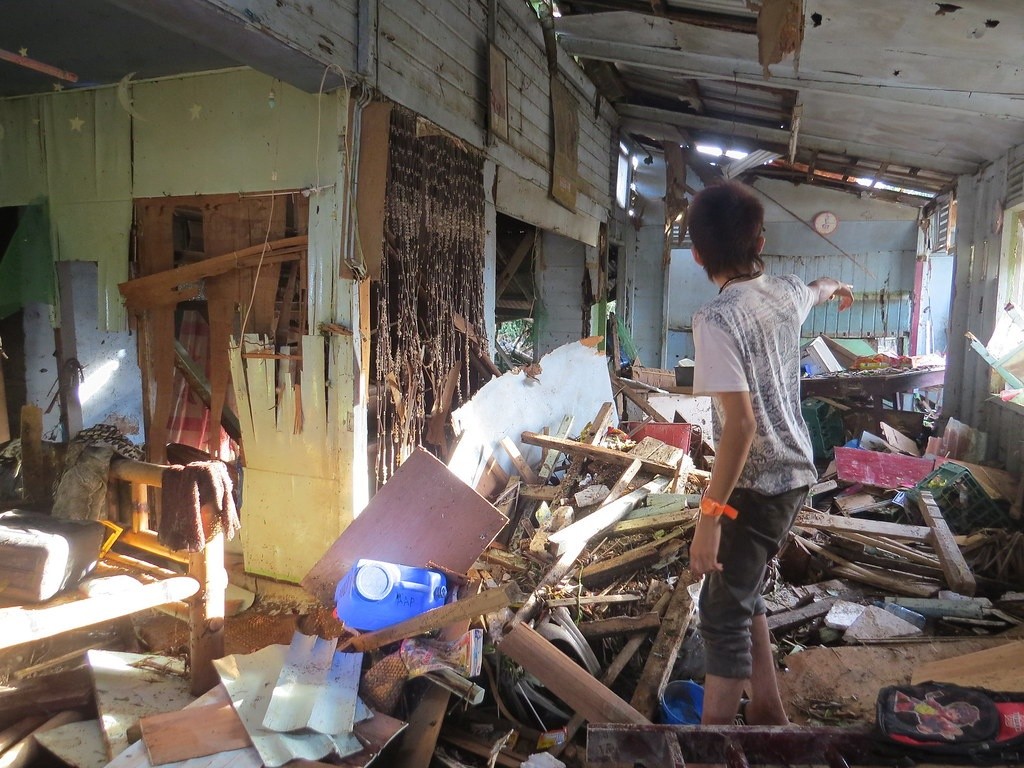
[814,211,839,235]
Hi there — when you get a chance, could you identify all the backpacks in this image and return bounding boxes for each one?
[875,680,1024,764]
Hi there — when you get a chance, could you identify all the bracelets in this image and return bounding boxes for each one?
[700,494,738,523]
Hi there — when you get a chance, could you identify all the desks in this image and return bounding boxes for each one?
[800,365,945,441]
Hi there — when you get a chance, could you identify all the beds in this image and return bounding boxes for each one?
[0,406,227,698]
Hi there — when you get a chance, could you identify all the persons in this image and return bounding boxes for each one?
[682,180,854,726]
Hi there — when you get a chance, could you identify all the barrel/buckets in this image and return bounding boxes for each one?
[660,681,705,726]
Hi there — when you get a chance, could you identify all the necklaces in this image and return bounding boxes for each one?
[718,270,762,297]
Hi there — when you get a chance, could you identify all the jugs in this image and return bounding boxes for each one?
[334,560,447,633]
